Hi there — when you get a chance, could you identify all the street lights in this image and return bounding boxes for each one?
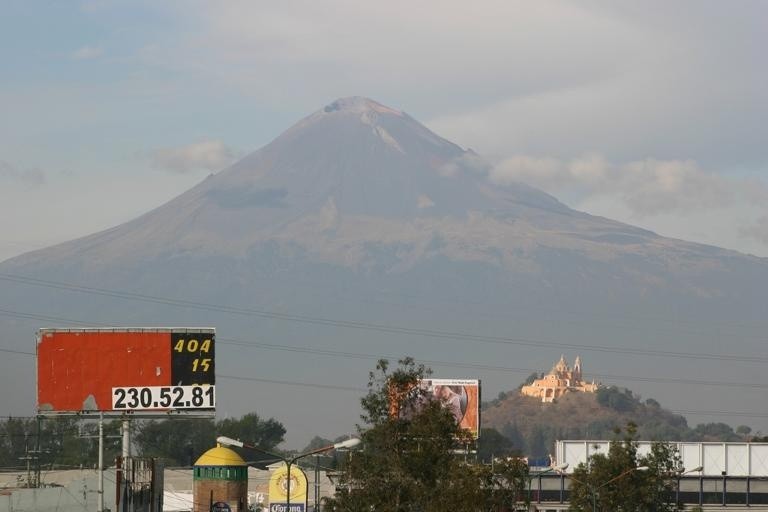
[675,466,703,510]
[550,466,649,512]
[215,434,361,512]
[522,463,568,512]
